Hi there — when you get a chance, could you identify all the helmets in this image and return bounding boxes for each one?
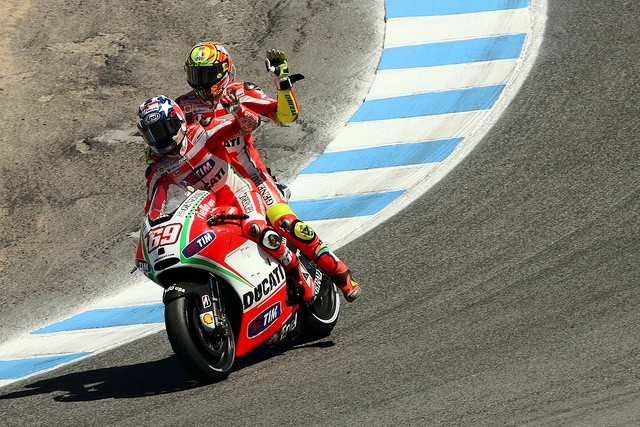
[137,94,187,158]
[185,41,232,101]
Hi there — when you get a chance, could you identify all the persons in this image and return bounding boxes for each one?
[176,41,363,302]
[127,95,315,302]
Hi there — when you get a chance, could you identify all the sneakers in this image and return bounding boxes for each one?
[331,269,363,302]
[288,259,314,301]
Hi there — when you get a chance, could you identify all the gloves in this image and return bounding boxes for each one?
[265,48,305,90]
[219,93,240,113]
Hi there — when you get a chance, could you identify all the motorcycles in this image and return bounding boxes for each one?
[130,167,341,387]
